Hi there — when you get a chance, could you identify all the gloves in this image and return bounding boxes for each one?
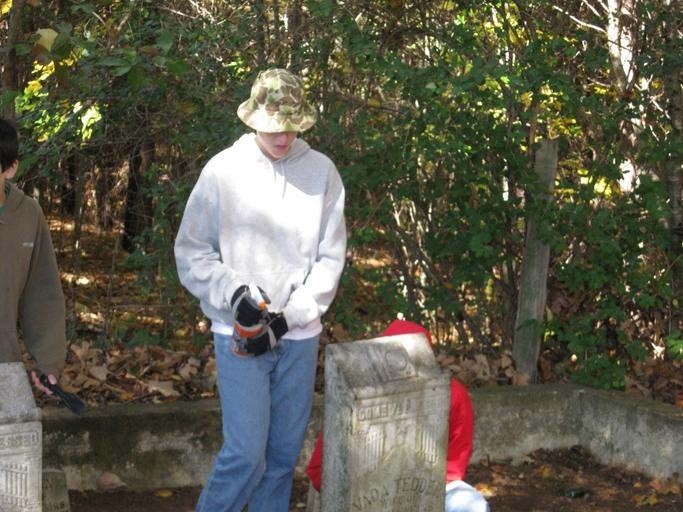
[242,313,288,357]
[230,284,271,326]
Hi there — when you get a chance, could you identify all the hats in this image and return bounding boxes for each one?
[237,69,319,134]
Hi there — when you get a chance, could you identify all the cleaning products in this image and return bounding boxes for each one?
[229,282,267,357]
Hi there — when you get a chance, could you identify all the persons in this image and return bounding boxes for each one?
[300,314,491,510]
[0,113,71,399]
[169,66,347,510]
